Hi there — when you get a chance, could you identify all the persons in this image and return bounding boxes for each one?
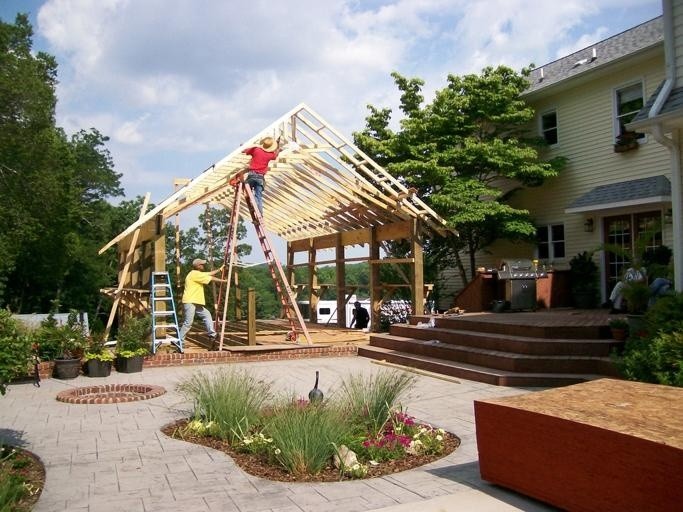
[348,302,369,330]
[602,258,645,314]
[177,257,229,348]
[237,136,285,216]
[647,255,675,304]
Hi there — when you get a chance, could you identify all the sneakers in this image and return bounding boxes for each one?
[608,307,621,314]
[209,333,221,342]
[601,300,614,309]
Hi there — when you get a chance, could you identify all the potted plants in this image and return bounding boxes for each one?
[590,215,671,313]
[117,346,148,372]
[40,322,90,379]
[83,351,115,377]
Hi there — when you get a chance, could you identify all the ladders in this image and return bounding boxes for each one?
[207,171,313,352]
[152,271,184,355]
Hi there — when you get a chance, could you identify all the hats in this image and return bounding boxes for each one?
[193,258,206,266]
[259,136,278,152]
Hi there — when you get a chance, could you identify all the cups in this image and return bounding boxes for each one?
[533,259,539,271]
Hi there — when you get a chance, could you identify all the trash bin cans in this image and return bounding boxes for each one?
[497,256,547,312]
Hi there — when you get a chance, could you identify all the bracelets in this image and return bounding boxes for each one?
[218,268,223,273]
